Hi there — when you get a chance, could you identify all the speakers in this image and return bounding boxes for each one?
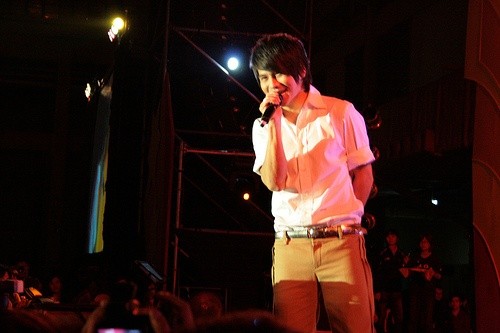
[166,0,313,313]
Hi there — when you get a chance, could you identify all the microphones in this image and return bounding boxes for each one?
[260,94,283,127]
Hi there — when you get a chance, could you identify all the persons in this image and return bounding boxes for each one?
[250,32,377,333]
[0,261,288,333]
[373,229,477,333]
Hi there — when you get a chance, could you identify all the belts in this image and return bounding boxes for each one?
[275,224,363,238]
